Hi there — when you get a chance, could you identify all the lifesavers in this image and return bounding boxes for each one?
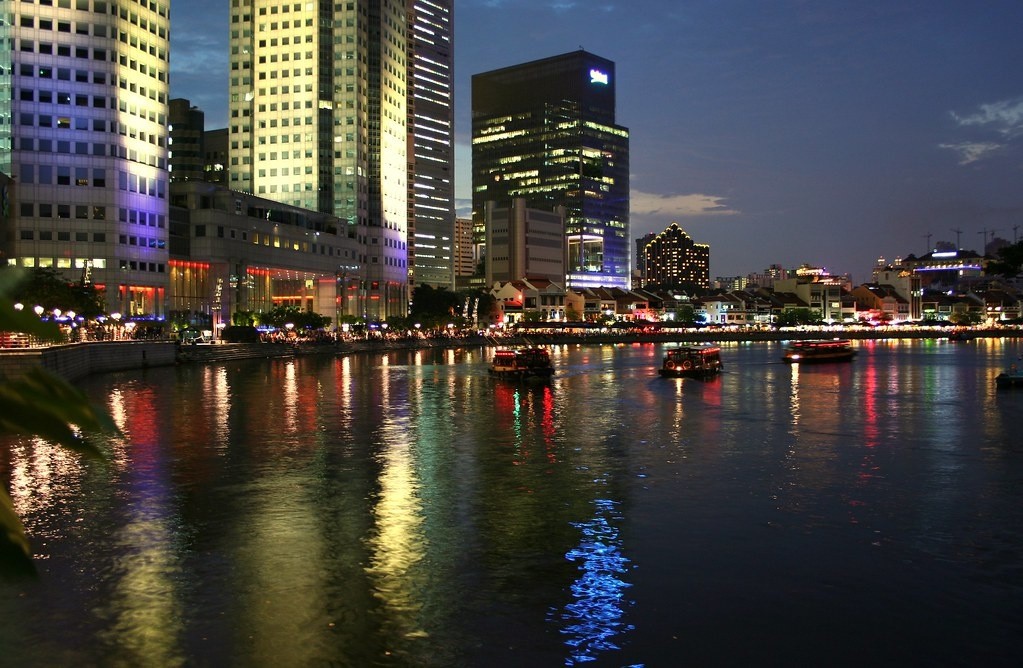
[666,360,675,369]
[683,361,692,369]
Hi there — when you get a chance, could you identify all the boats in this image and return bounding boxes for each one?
[780,337,862,365]
[658,343,724,380]
[486,338,557,383]
[995,363,1023,390]
